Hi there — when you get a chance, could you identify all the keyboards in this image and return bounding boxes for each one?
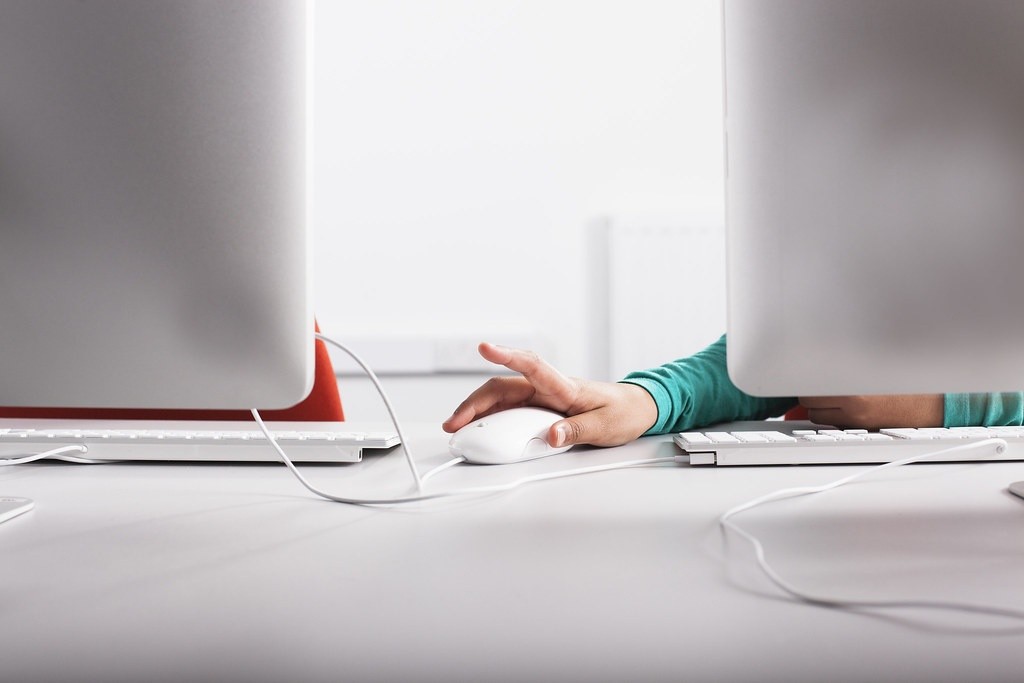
[0,429,403,462]
[673,426,1024,465]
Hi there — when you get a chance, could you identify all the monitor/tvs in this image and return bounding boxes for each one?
[0,0,317,409]
[717,0,1024,402]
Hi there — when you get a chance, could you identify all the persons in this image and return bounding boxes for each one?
[442,333,1024,449]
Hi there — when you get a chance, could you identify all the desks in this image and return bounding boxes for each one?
[0,420,1024,683]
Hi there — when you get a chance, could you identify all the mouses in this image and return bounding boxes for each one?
[447,405,576,465]
